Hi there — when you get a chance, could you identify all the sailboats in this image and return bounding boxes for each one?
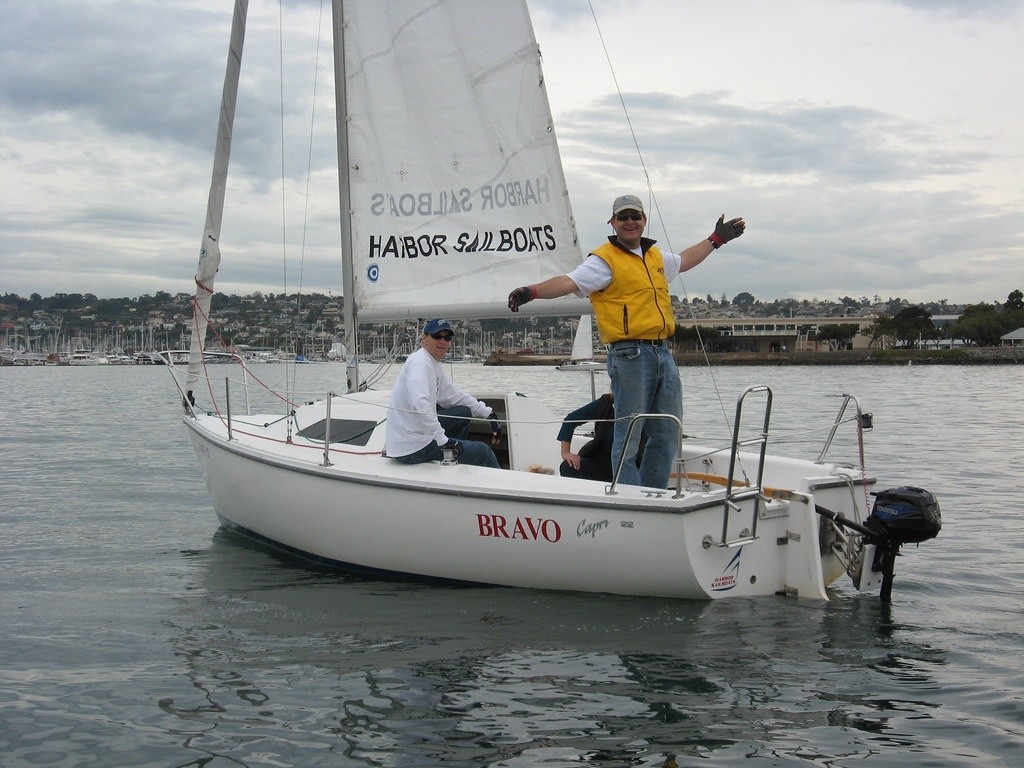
[159,0,945,628]
[0,312,485,389]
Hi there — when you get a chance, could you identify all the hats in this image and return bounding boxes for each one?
[607,194,644,225]
[423,317,454,337]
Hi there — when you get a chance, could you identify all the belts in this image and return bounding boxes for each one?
[626,339,663,346]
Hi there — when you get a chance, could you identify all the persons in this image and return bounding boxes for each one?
[557,393,649,482]
[385,318,503,469]
[508,194,745,491]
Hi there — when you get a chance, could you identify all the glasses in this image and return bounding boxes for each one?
[429,332,452,341]
[614,213,643,221]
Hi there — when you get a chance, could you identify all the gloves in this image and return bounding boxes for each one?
[439,437,464,460]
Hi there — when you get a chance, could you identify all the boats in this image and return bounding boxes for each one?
[555,315,609,371]
